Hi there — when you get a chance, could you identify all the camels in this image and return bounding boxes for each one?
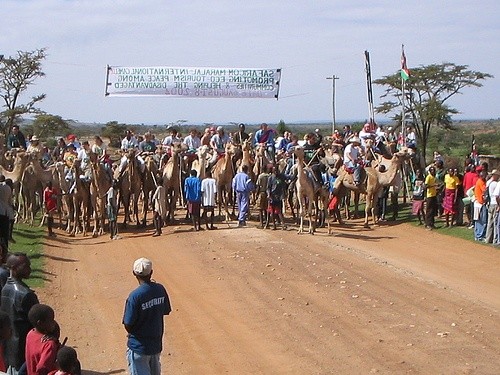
[0,144,413,239]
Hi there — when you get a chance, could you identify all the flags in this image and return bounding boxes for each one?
[401,49,411,81]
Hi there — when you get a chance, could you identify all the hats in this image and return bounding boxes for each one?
[132,258,152,277]
[349,136,361,143]
[489,169,500,175]
[217,126,225,131]
[81,141,88,145]
[29,135,39,142]
[66,144,77,151]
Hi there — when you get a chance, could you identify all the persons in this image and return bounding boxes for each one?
[47,346,81,375]
[18,320,60,375]
[122,257,171,375]
[0,265,10,372]
[0,125,499,264]
[0,252,39,375]
[26,304,60,375]
